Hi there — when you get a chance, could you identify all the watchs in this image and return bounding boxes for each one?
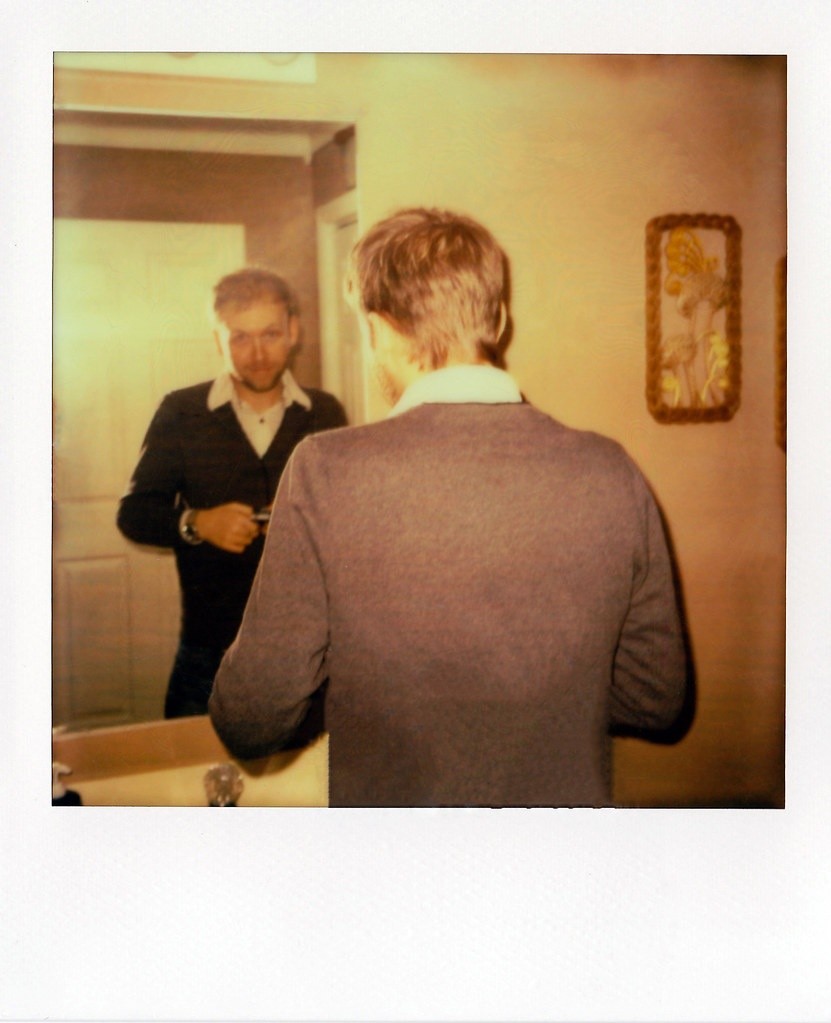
[182,510,202,544]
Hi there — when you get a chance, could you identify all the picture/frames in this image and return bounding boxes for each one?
[645,213,744,426]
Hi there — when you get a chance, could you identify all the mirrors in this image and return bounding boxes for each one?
[52,65,381,786]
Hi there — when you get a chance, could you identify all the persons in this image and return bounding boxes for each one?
[116,268,348,720]
[207,209,687,808]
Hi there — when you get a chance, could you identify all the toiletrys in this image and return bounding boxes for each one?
[52,760,84,807]
